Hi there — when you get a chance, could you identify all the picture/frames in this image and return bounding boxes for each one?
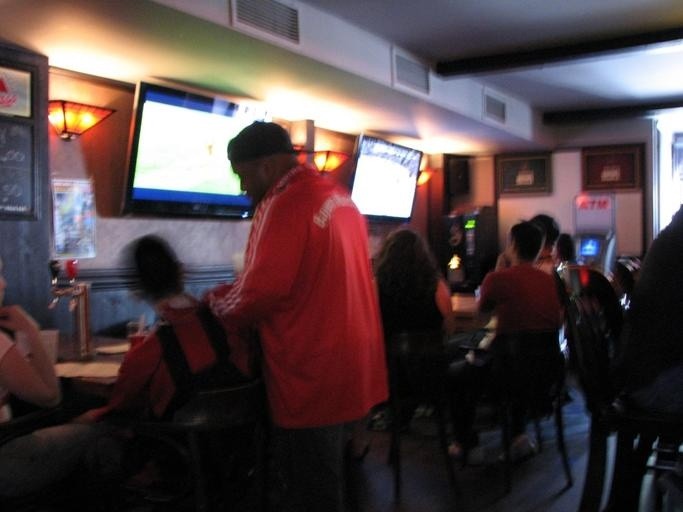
[493,152,551,194]
[579,143,646,191]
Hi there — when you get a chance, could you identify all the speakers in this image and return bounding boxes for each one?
[448,159,470,195]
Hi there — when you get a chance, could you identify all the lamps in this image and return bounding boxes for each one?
[48,98,116,144]
[297,149,350,174]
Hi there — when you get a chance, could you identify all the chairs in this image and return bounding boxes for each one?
[353,325,574,511]
[554,253,682,511]
[0,375,268,512]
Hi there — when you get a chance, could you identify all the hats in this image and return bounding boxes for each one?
[227,120,317,163]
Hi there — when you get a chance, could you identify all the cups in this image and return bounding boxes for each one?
[128,334,147,348]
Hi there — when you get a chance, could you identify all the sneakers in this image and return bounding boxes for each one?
[442,434,537,465]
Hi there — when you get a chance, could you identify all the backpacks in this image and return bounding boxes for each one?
[163,359,263,478]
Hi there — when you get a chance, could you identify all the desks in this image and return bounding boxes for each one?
[51,333,140,388]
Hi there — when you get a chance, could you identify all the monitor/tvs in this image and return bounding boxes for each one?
[580,239,600,257]
[121,80,268,220]
[349,134,423,223]
